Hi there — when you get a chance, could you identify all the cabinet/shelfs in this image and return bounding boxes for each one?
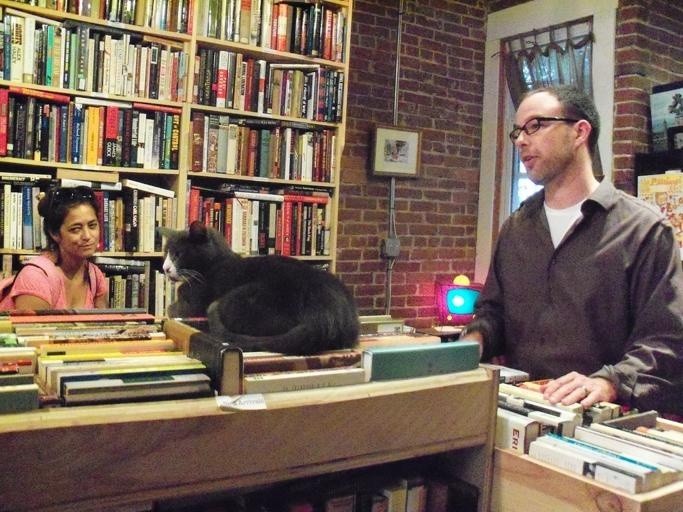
[0,362,501,512]
[0,0,354,325]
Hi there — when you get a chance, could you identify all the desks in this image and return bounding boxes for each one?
[413,325,465,345]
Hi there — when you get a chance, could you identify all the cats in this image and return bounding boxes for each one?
[154,220,363,355]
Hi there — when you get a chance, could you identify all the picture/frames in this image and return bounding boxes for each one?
[370,123,423,177]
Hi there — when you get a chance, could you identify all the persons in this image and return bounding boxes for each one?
[1,186,109,313]
[453,84,683,413]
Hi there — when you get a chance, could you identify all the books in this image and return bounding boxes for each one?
[1,257,179,325]
[0,307,479,412]
[2,0,196,102]
[1,86,179,170]
[486,363,683,497]
[637,173,683,262]
[186,113,330,255]
[650,88,682,152]
[1,168,180,251]
[230,467,480,511]
[193,1,349,123]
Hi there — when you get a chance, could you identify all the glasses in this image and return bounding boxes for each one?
[50,188,92,210]
[510,117,578,144]
